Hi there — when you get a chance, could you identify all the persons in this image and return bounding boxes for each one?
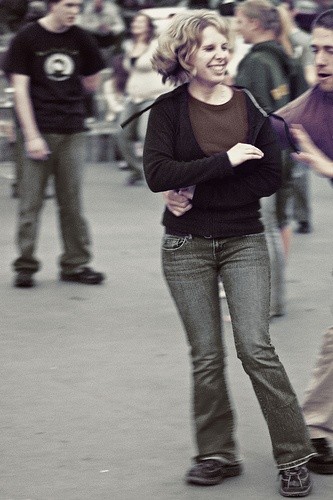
[0,0,333,473]
[120,9,313,496]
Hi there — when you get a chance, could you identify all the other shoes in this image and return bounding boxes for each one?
[185,458,241,485]
[309,438,333,474]
[277,464,312,498]
[60,267,103,284]
[15,271,32,286]
